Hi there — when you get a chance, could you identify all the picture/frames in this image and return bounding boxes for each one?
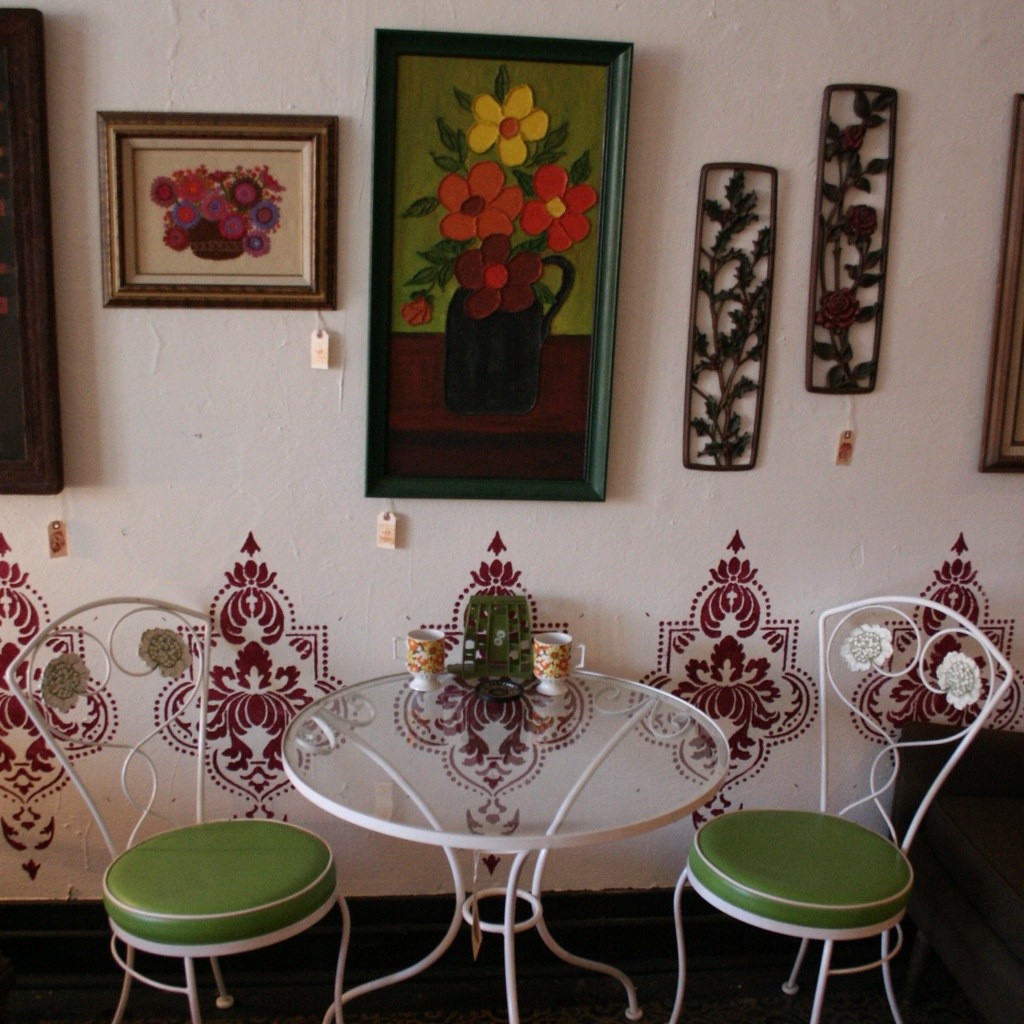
[0,8,66,494]
[96,107,342,322]
[354,27,637,503]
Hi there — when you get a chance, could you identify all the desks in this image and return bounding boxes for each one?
[280,663,732,1024]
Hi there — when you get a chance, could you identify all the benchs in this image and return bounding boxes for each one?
[890,721,1024,1024]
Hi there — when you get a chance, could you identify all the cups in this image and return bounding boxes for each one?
[393,628,445,691]
[533,632,586,696]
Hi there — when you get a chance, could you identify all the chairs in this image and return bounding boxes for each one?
[663,595,1016,1024]
[5,597,350,1024]
